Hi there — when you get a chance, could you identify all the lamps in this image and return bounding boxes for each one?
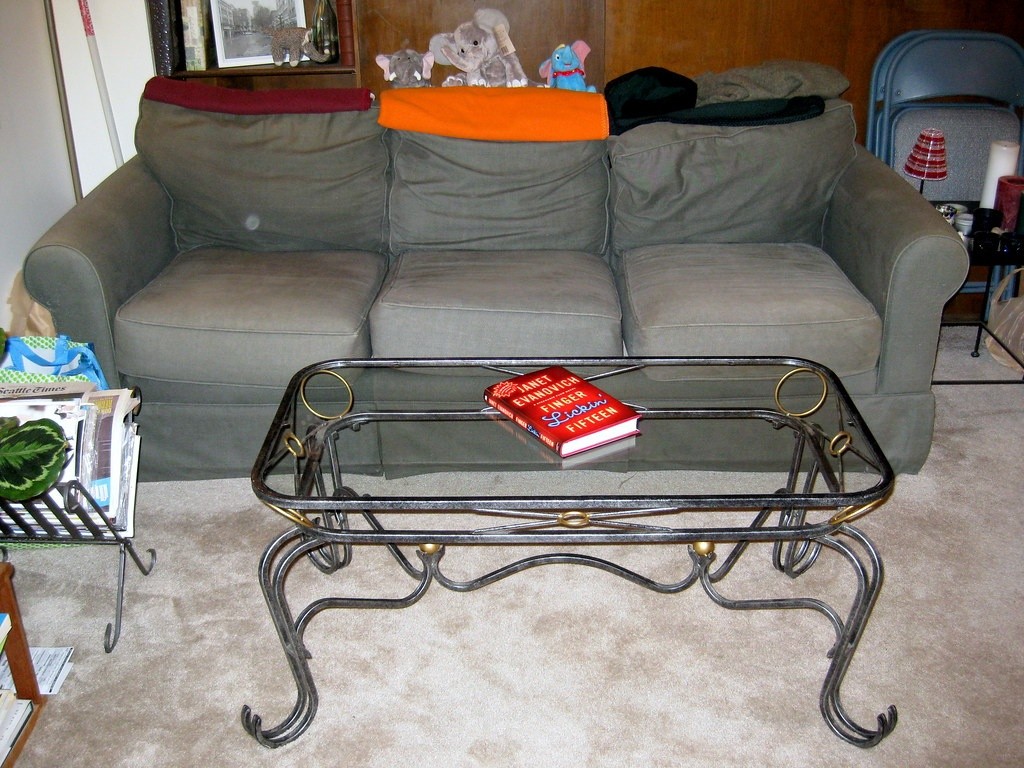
[904,124,950,194]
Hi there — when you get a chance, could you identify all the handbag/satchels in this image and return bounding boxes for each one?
[0,334,110,552]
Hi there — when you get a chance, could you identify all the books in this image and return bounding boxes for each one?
[483,366,643,458]
[1,612,34,766]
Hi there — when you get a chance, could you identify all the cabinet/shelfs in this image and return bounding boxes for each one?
[169,0,362,94]
[1,562,46,768]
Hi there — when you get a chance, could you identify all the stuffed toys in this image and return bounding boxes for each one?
[429,9,545,88]
[375,49,434,89]
[539,40,596,93]
[268,28,330,67]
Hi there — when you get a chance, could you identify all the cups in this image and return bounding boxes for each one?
[956,214,972,235]
[946,204,967,214]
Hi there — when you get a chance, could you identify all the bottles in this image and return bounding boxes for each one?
[311,0,339,63]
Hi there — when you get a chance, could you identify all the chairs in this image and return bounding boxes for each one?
[866,28,1024,320]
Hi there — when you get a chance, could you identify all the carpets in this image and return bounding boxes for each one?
[0,325,1024,767]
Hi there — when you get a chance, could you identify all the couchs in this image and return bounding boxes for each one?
[21,73,969,480]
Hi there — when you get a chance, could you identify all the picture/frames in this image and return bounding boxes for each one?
[211,1,310,69]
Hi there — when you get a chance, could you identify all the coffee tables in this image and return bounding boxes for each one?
[240,353,902,749]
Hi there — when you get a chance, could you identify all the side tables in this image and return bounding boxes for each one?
[928,199,1024,385]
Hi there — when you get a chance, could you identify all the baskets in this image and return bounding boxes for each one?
[984,267,1024,373]
